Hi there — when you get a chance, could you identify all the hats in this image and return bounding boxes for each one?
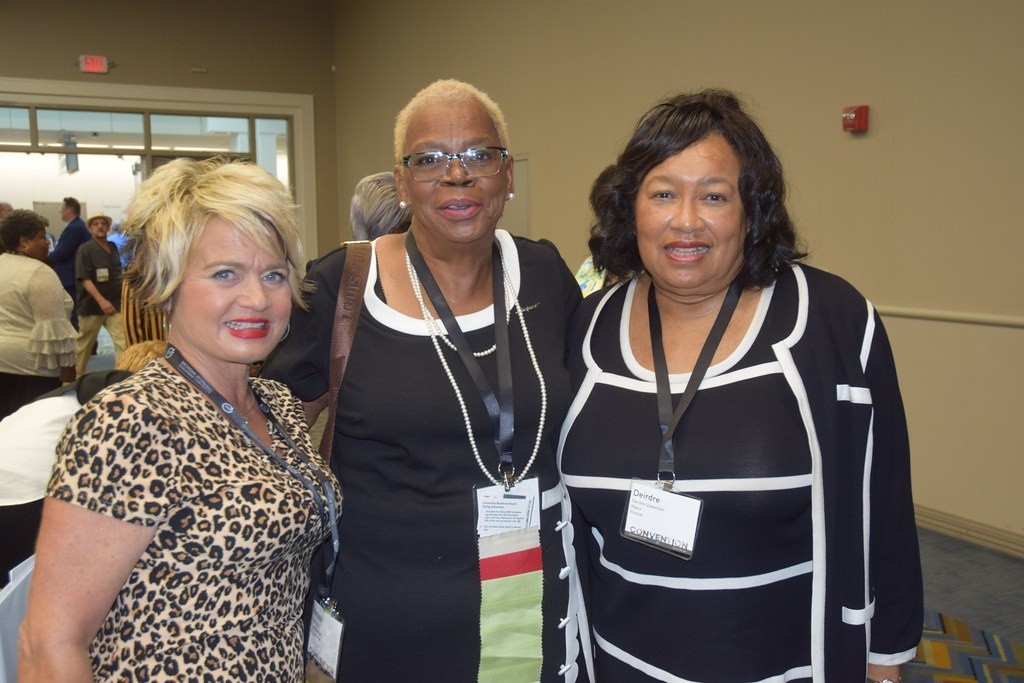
[86,211,112,230]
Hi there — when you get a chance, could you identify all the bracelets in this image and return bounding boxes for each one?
[867,677,903,683]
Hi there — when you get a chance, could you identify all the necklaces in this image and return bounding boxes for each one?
[239,388,254,419]
[406,253,546,487]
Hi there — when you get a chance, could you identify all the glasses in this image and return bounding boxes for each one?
[403,146,509,183]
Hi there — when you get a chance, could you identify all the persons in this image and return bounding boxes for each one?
[18,158,343,683]
[260,79,584,683]
[0,197,126,422]
[554,87,926,683]
